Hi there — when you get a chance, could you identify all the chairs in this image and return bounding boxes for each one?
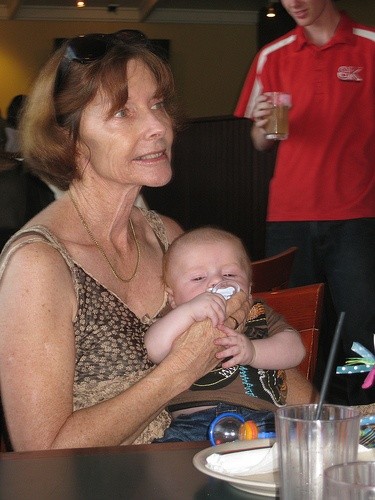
[249,246,299,295]
[251,282,326,384]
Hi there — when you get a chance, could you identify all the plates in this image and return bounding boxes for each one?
[191,439,375,498]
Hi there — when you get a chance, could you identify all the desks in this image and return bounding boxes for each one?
[0,437,238,500]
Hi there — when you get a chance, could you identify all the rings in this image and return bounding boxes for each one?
[230,316,238,328]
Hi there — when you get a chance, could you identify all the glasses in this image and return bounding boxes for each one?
[53,29,152,128]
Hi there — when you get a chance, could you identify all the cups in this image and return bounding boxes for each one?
[263,90,293,142]
[321,459,374,499]
[273,405,361,499]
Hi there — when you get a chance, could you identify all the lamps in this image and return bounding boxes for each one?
[267,3,276,18]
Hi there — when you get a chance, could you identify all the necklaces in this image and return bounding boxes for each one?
[68,190,139,282]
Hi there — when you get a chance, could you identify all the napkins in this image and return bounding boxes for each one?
[204,437,370,478]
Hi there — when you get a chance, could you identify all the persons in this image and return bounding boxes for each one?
[233,0,375,408]
[0,27,254,453]
[144,228,306,443]
[0,116,26,252]
